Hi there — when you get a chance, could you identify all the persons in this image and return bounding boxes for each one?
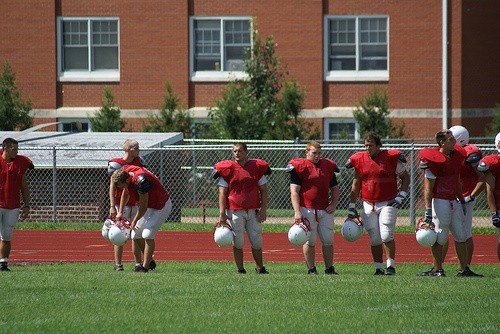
[0,137,35,272]
[284,141,340,275]
[417,129,484,277]
[441,124,487,272]
[111,164,172,273]
[212,141,273,274]
[345,132,410,275]
[108,140,147,271]
[477,131,500,265]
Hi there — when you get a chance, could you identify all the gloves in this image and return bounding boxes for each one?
[491,211,500,228]
[388,191,408,208]
[425,208,432,223]
[348,203,358,219]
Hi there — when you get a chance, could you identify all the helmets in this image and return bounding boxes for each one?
[288,222,312,246]
[341,222,364,241]
[102,219,114,240]
[449,126,469,147]
[494,133,500,152]
[214,225,233,247]
[415,228,437,249]
[108,225,133,246]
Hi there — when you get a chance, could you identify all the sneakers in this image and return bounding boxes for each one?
[458,266,483,277]
[308,267,319,274]
[114,264,124,271]
[238,269,247,274]
[374,268,384,275]
[139,267,148,272]
[133,265,142,272]
[255,266,269,274]
[325,266,338,274]
[148,260,156,270]
[0,262,10,271]
[420,268,443,276]
[385,266,395,275]
